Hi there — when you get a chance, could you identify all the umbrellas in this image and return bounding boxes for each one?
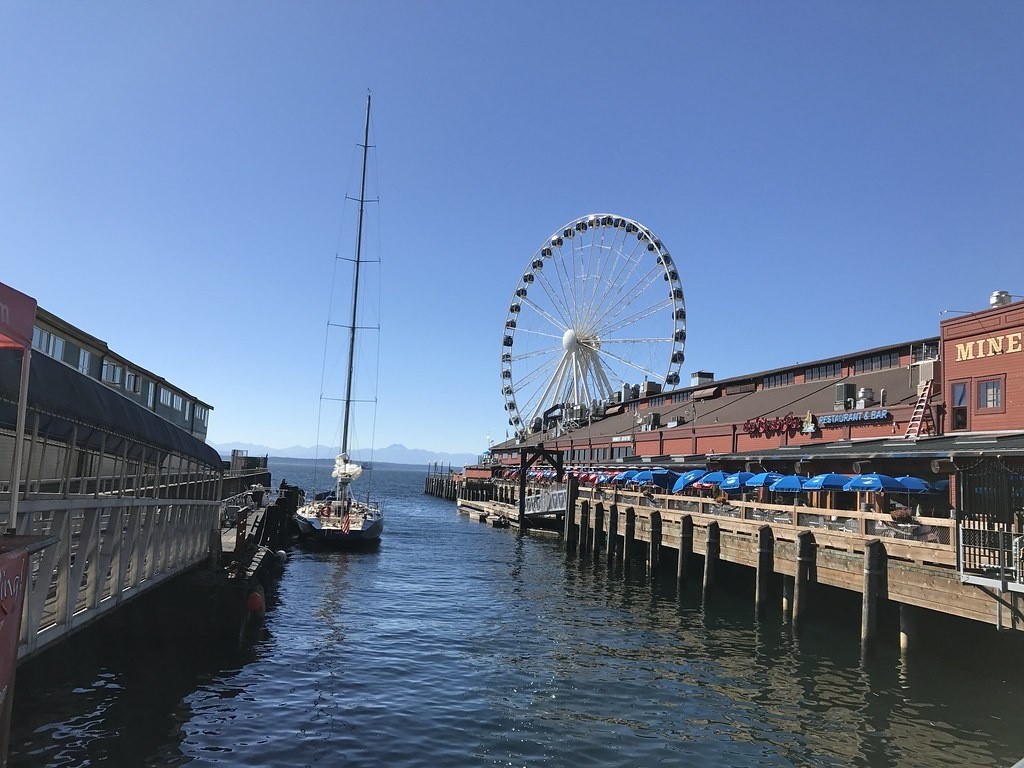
[719,471,757,495]
[672,470,709,493]
[632,471,654,482]
[769,474,809,492]
[745,471,787,486]
[802,472,851,491]
[698,470,732,485]
[503,468,622,484]
[651,469,682,490]
[611,470,639,484]
[843,472,939,494]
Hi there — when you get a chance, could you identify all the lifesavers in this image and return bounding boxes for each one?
[320,506,331,517]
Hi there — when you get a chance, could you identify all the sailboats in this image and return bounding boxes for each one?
[292,89,386,544]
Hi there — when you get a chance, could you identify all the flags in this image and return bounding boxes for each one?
[341,511,350,534]
